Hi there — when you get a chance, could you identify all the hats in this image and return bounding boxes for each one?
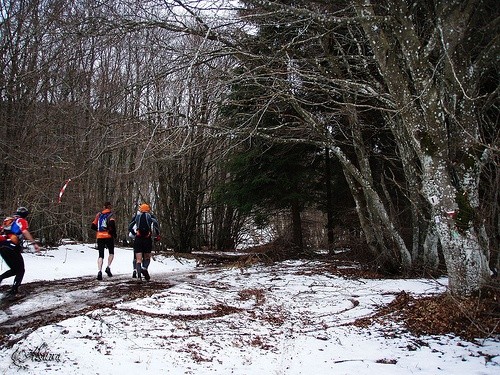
[16,207,29,217]
[140,204,151,213]
[103,201,113,209]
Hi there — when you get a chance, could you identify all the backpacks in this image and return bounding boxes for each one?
[98,212,113,231]
[0,215,24,241]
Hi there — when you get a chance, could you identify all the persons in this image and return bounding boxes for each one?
[127,204,161,280]
[90,201,119,280]
[0,206,42,297]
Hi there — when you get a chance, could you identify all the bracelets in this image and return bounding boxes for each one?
[32,241,37,245]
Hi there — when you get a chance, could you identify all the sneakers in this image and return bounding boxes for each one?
[7,290,26,301]
[133,269,151,281]
[97,267,113,280]
